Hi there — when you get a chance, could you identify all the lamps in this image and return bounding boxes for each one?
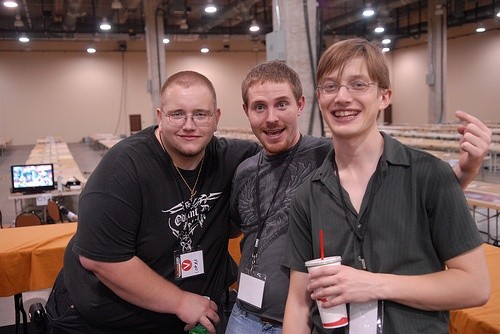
[250,18,260,32]
[111,0,122,9]
[3,0,19,7]
[85,43,98,53]
[20,32,29,43]
[474,21,486,32]
[204,0,216,12]
[363,2,392,52]
[14,13,25,27]
[100,16,112,30]
[201,43,210,52]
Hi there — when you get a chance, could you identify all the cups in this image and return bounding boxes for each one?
[305,255,349,329]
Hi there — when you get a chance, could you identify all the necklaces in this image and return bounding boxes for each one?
[165,149,205,200]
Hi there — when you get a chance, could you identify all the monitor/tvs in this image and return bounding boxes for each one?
[11,164,54,195]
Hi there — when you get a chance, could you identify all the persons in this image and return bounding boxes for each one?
[44,71,264,334]
[281,37,490,334]
[224,62,493,334]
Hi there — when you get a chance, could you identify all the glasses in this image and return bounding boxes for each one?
[317,78,381,95]
[163,109,215,127]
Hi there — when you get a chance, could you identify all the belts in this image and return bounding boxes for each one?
[258,316,283,326]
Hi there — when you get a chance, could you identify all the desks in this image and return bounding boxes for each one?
[0,132,126,224]
[0,222,78,334]
[216,123,499,333]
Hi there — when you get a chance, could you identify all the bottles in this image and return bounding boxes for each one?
[55,201,77,222]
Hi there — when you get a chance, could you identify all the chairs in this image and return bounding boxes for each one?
[16,213,44,226]
[49,200,69,224]
